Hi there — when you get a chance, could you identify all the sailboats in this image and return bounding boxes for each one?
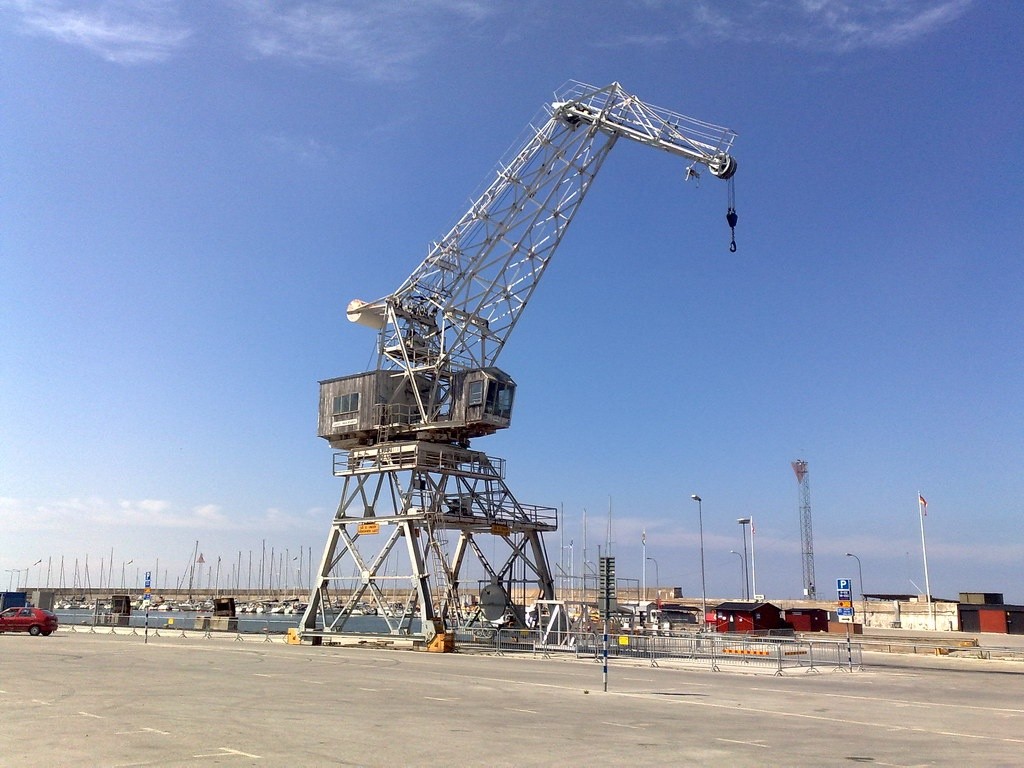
[52,540,440,619]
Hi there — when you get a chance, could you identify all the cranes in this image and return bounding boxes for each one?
[286,78,738,654]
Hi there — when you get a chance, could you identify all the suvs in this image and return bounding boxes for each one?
[0,607,58,637]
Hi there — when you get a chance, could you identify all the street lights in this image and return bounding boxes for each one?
[691,494,706,632]
[730,550,744,601]
[587,560,598,602]
[646,557,659,600]
[737,516,751,601]
[845,552,867,625]
